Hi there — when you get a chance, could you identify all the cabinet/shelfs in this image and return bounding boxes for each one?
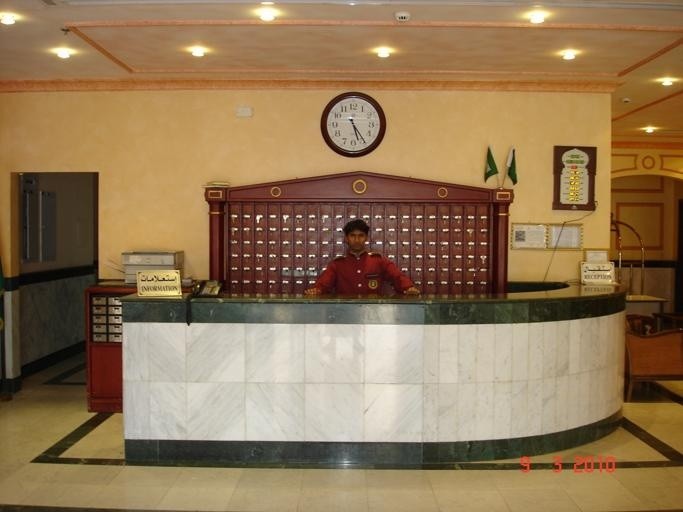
[82,281,198,415]
[203,169,513,294]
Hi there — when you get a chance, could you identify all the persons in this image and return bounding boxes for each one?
[302,219,421,297]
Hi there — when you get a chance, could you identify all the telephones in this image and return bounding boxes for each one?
[193,280,223,298]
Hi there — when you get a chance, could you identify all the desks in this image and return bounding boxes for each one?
[623,293,668,317]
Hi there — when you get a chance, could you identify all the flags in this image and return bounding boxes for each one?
[483,145,498,183]
[506,144,518,186]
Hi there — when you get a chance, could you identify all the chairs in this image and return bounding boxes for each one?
[624,314,681,401]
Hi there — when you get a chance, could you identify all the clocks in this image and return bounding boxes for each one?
[318,91,387,158]
